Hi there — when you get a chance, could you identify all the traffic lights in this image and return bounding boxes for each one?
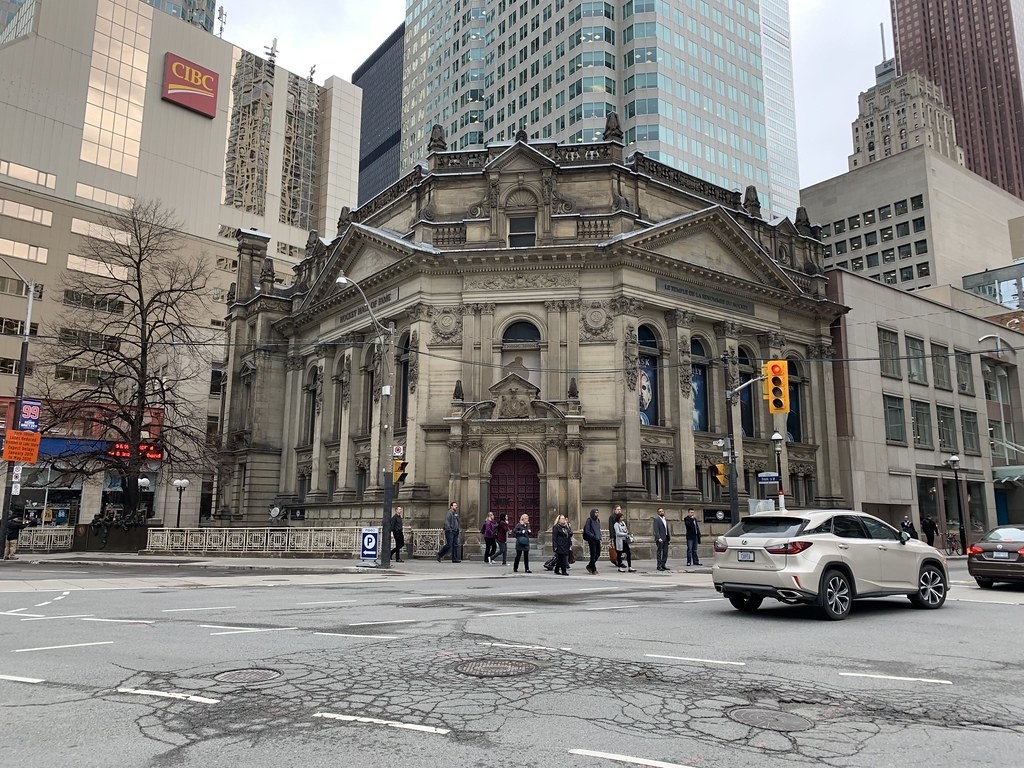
[391,459,408,485]
[709,464,726,487]
[767,359,792,414]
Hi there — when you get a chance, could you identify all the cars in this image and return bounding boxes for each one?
[711,508,952,622]
[966,522,1024,589]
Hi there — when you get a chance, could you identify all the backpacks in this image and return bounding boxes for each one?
[903,521,912,534]
[583,518,591,541]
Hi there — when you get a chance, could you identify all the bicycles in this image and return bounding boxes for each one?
[944,532,963,557]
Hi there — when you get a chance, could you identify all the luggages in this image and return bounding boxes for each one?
[544,554,558,570]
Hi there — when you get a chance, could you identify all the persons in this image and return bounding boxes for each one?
[552,514,574,575]
[482,512,497,564]
[653,508,671,572]
[514,514,532,573]
[390,506,405,562]
[436,502,462,563]
[488,513,512,565]
[901,516,918,540]
[4,510,37,560]
[583,508,603,575]
[608,504,637,573]
[683,508,704,566]
[922,514,941,547]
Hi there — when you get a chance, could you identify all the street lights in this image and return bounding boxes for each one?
[137,478,151,510]
[337,269,396,570]
[173,478,189,528]
[770,430,787,510]
[949,453,967,555]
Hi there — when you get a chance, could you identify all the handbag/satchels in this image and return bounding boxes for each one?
[481,522,486,534]
[626,535,634,543]
[518,536,529,545]
[608,546,617,560]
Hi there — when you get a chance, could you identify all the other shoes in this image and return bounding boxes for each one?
[525,570,532,573]
[562,571,569,576]
[488,556,492,565]
[513,569,518,573]
[687,562,690,566]
[390,552,392,560]
[554,569,561,574]
[694,562,702,566]
[502,563,511,566]
[491,559,497,564]
[452,560,461,563]
[436,553,441,562]
[663,566,670,570]
[657,566,665,571]
[612,561,636,572]
[586,566,599,575]
[396,559,404,562]
